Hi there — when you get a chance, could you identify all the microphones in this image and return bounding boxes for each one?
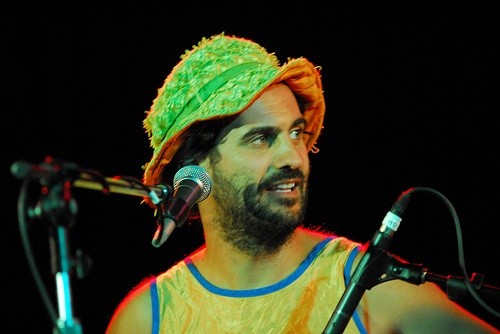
[322,190,412,334]
[152,165,212,248]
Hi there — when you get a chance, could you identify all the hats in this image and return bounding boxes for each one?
[144,35,325,219]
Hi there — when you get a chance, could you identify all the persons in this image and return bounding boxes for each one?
[105,32,500,334]
